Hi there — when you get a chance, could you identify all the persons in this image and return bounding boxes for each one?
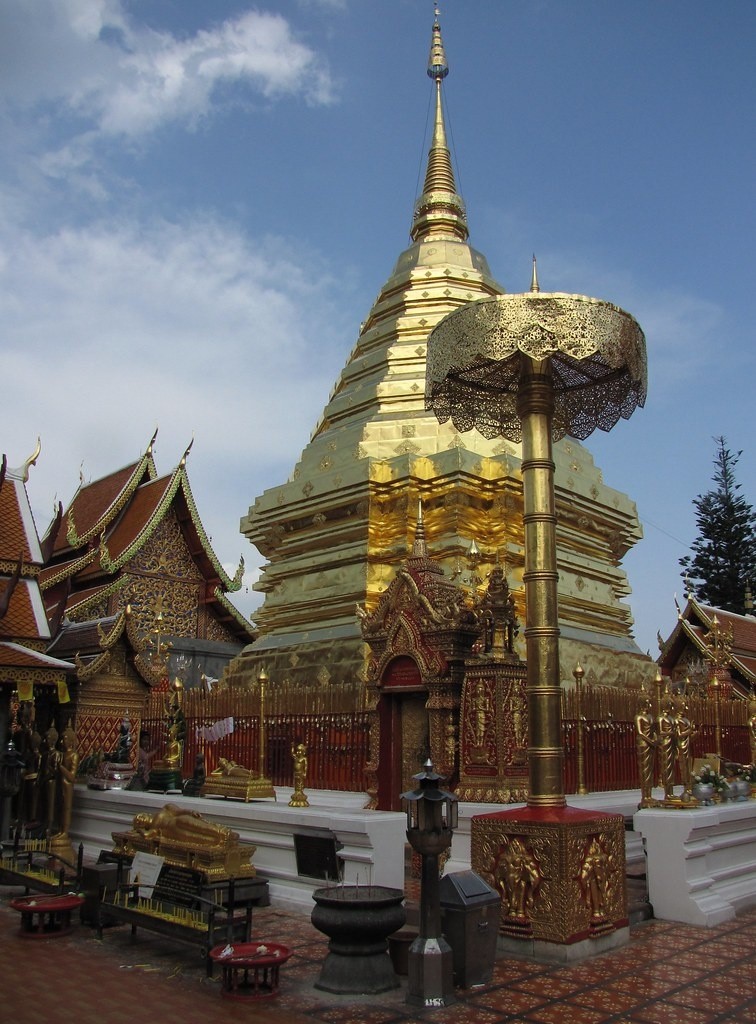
[636,682,696,800]
[105,703,189,767]
[18,717,79,839]
[187,754,206,785]
[747,694,756,766]
[132,803,240,846]
[211,757,257,779]
[291,742,308,793]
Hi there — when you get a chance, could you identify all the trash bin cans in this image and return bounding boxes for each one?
[439,868,503,990]
